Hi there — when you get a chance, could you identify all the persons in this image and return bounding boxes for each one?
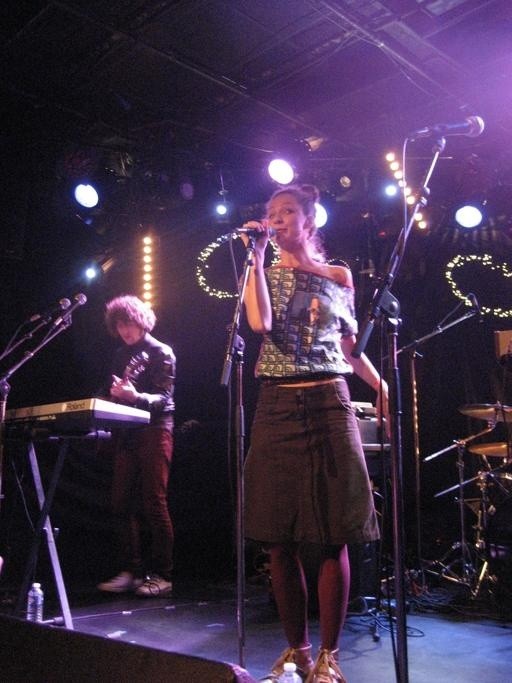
[240,185,392,683]
[94,293,177,600]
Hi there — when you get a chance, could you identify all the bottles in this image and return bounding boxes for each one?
[26,582,45,618]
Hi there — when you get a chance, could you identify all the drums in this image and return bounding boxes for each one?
[477,472,512,546]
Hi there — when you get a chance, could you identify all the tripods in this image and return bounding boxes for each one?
[340,316,406,643]
[424,462,474,586]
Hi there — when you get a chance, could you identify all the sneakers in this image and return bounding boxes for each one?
[134,572,173,596]
[314,648,340,683]
[257,646,314,683]
[96,570,144,592]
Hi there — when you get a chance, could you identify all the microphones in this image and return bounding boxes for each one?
[235,226,276,237]
[54,294,87,326]
[27,298,71,323]
[408,116,486,141]
[468,293,484,324]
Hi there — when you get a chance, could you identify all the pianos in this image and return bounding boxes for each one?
[0,398,151,435]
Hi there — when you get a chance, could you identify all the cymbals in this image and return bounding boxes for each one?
[457,404,512,424]
[469,442,512,458]
